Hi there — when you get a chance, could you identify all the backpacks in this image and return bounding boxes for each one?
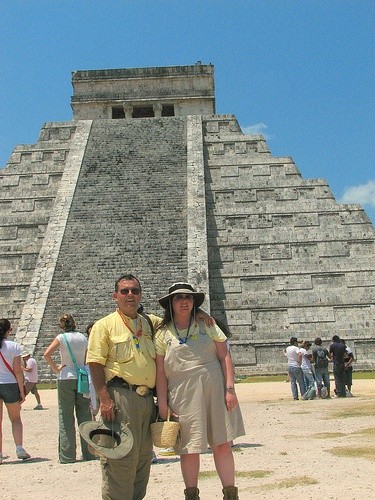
[314,347,328,368]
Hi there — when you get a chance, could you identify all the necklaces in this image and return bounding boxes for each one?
[174,316,191,344]
[119,312,143,349]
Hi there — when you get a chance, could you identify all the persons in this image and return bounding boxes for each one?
[340,339,354,393]
[299,340,316,399]
[86,274,214,500]
[330,335,347,398]
[215,317,230,354]
[286,337,308,400]
[86,323,100,416]
[312,338,332,399]
[21,351,43,410]
[154,281,245,500]
[44,314,95,464]
[0,318,30,463]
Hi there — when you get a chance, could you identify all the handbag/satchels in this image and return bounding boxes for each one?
[321,385,328,399]
[77,368,90,393]
[150,406,179,448]
[302,385,315,400]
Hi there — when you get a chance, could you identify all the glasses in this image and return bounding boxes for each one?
[117,288,140,295]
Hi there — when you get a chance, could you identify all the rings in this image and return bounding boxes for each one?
[112,412,115,414]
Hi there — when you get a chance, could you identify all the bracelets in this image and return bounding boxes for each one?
[347,363,349,365]
[226,387,234,391]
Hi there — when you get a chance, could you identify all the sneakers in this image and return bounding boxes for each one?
[16,448,31,459]
[0,456,2,464]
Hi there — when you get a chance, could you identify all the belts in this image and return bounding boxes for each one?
[108,380,154,396]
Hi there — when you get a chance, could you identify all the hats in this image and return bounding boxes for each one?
[21,351,30,357]
[157,282,205,309]
[78,420,134,459]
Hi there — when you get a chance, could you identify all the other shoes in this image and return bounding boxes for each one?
[151,457,157,462]
[184,487,200,500]
[159,447,176,457]
[222,485,239,500]
[334,395,340,398]
[34,404,43,410]
